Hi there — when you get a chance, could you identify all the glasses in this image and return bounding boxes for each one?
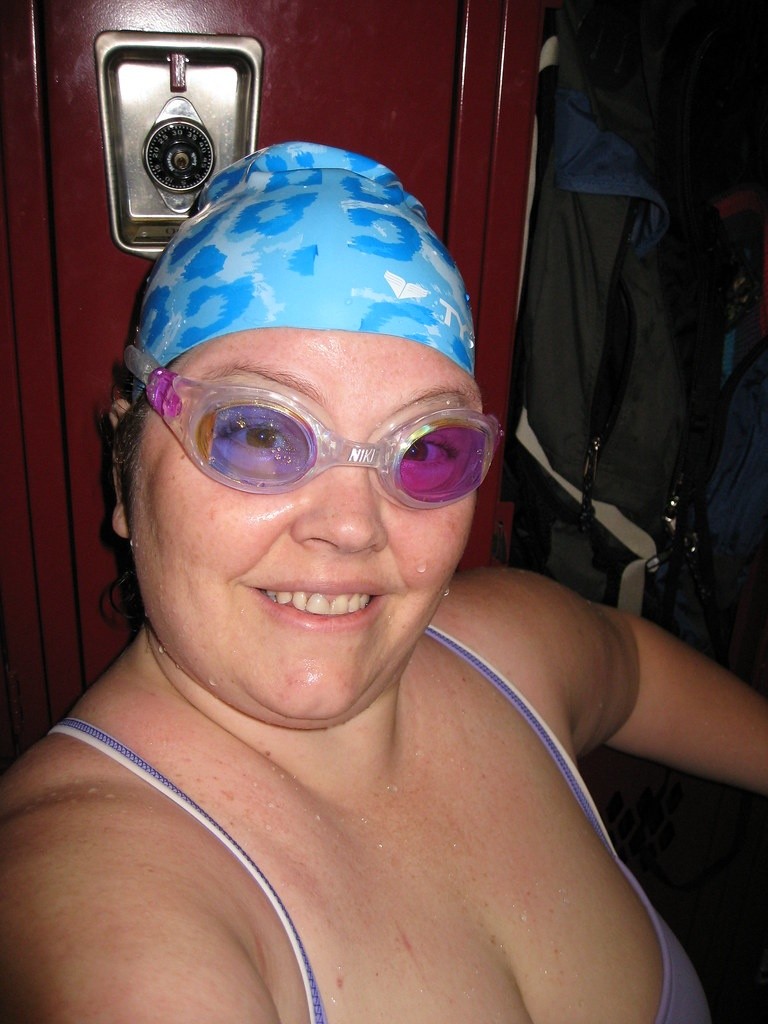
[124,344,501,509]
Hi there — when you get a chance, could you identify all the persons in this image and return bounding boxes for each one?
[0,140,768,1024]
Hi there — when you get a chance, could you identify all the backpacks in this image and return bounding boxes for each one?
[528,5,682,599]
